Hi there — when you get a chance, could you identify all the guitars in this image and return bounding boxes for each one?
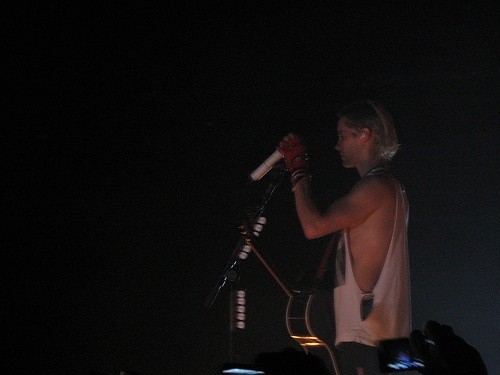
[237,223,341,375]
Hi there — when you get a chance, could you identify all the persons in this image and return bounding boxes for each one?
[276,97,410,375]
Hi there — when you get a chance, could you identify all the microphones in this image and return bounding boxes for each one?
[248,133,295,183]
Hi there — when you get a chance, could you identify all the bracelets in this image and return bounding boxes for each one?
[275,139,313,192]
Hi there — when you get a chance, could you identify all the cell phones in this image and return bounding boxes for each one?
[377,336,440,373]
[222,363,265,375]
